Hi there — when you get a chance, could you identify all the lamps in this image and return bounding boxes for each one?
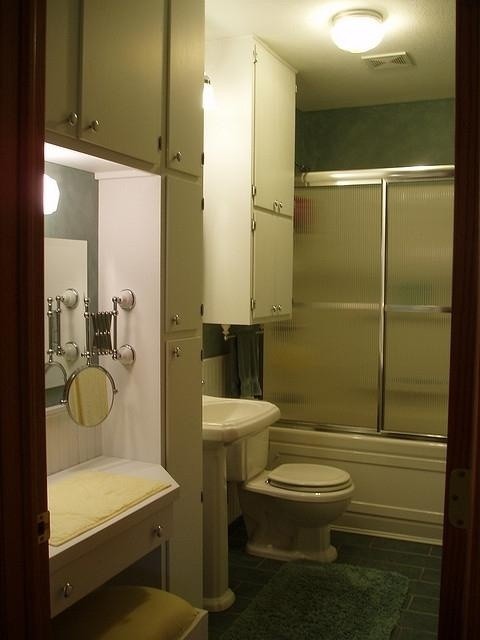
[330,9,384,53]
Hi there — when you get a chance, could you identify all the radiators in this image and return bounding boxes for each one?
[201,353,242,528]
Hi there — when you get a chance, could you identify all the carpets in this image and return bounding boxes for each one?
[219,557,409,640]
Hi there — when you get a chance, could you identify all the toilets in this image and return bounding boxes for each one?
[226,427,354,564]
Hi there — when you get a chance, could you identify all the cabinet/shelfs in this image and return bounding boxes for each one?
[203,33,296,326]
[44,0,206,622]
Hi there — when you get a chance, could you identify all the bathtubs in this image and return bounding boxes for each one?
[269,419,447,545]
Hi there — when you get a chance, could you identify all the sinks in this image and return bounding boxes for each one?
[200,393,281,443]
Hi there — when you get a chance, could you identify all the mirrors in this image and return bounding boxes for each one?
[61,291,134,428]
[44,288,80,409]
[43,160,98,409]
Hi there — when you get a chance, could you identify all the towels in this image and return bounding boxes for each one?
[47,473,172,548]
[229,331,263,398]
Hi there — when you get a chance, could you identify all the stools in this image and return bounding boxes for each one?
[52,585,208,640]
